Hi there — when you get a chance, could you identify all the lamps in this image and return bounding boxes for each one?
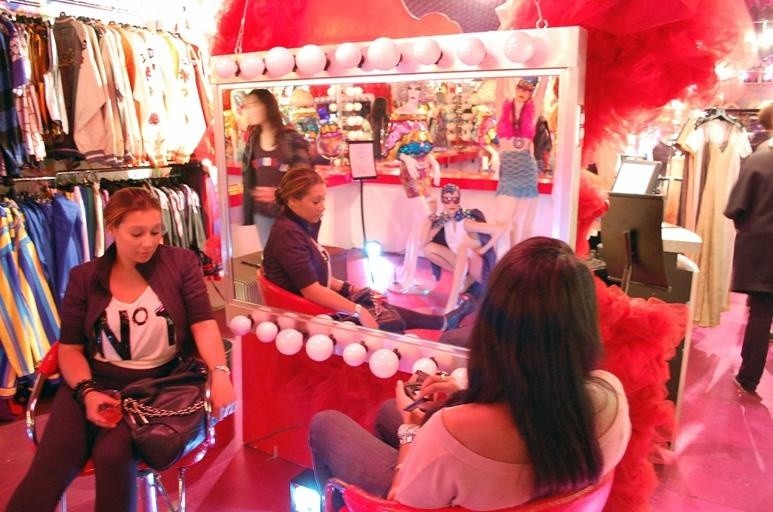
[228,313,467,389]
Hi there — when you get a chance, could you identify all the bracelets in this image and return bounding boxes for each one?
[210,365,231,373]
[71,379,99,398]
[341,281,350,295]
[396,423,419,445]
[352,304,362,319]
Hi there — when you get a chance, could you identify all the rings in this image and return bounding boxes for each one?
[440,370,448,380]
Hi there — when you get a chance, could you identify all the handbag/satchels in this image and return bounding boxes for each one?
[346,287,407,335]
[114,360,207,472]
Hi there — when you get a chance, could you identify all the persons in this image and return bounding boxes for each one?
[497,76,547,245]
[391,81,438,292]
[421,183,509,312]
[370,98,391,163]
[308,236,632,512]
[241,89,310,251]
[0,187,240,512]
[749,103,773,148]
[727,148,773,394]
[261,159,377,336]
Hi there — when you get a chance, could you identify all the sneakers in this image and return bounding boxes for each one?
[733,370,758,394]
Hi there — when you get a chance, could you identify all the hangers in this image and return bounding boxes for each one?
[207,26,586,393]
[694,96,749,133]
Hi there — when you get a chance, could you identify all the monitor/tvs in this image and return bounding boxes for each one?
[611,159,663,193]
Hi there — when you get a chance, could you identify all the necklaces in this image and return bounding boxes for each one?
[509,101,527,148]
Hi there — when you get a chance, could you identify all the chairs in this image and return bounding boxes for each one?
[249,263,341,317]
[23,315,234,512]
[320,467,620,512]
